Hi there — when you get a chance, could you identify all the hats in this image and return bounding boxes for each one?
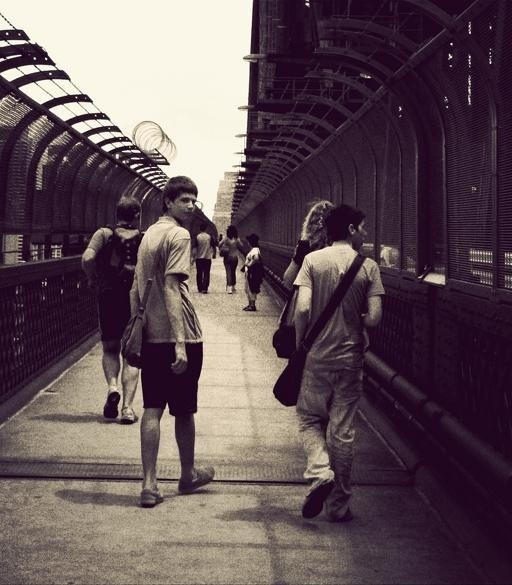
[246,234,259,247]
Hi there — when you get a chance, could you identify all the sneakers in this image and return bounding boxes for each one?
[179,465,215,492]
[301,479,333,518]
[121,408,135,424]
[141,489,163,506]
[104,386,120,418]
[328,508,352,521]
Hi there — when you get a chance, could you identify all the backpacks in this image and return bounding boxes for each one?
[95,225,143,296]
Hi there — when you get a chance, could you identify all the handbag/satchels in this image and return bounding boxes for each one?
[122,307,144,367]
[220,246,230,257]
[273,326,296,358]
[274,351,307,406]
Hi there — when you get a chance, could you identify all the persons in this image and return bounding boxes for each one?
[292,204,386,522]
[283,200,337,327]
[191,223,264,311]
[129,176,216,507]
[82,197,146,425]
